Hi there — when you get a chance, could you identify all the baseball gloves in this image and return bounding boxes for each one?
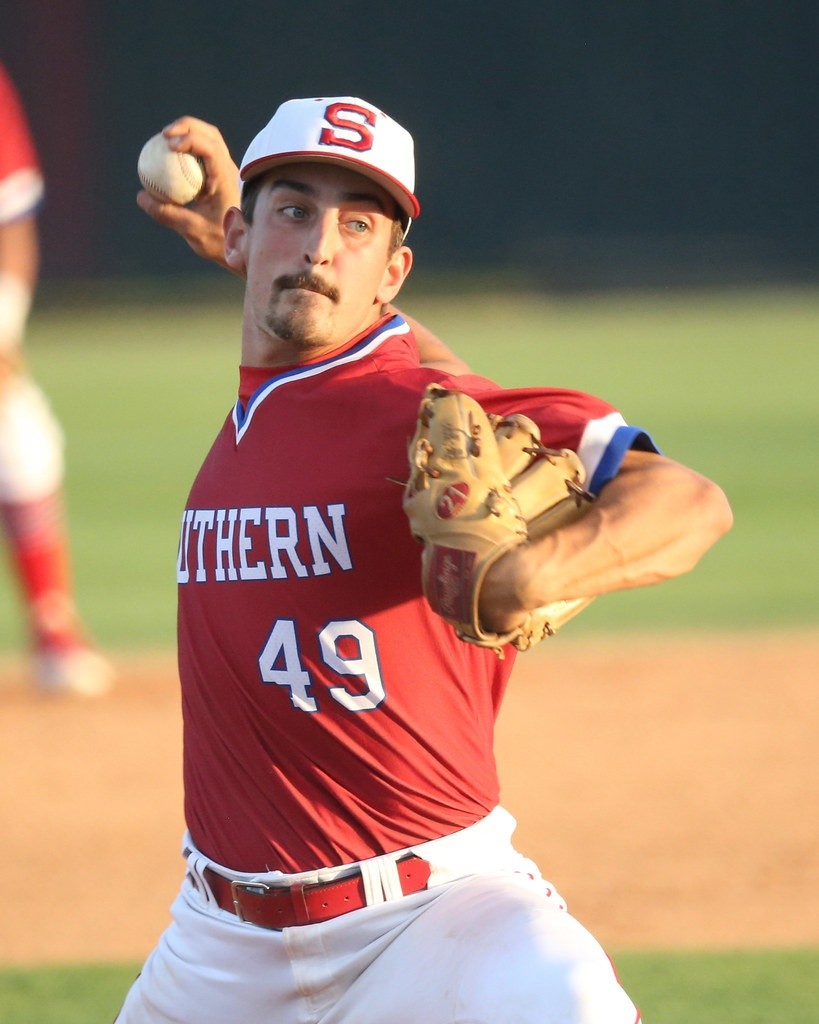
[383,380,600,662]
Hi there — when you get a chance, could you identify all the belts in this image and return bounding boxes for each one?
[183,850,430,932]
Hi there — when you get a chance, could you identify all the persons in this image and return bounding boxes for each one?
[98,96,733,1024]
[0,57,111,695]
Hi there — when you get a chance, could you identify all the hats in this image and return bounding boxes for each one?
[239,95,422,221]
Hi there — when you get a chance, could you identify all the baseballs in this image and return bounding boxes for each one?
[136,130,207,207]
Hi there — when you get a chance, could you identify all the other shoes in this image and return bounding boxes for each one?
[27,643,111,692]
